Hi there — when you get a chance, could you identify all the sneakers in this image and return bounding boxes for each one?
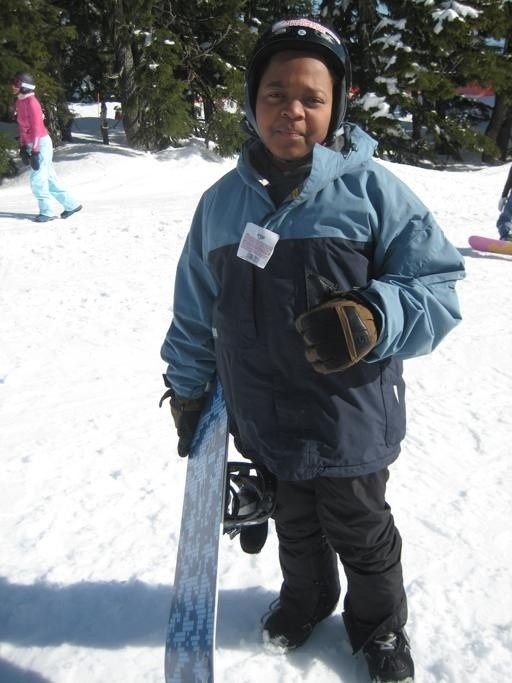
[360,632,416,683]
[60,204,83,218]
[32,214,59,222]
[259,584,340,655]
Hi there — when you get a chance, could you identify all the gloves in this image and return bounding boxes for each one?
[496,195,507,210]
[20,146,32,166]
[30,150,41,170]
[157,371,208,460]
[293,287,386,382]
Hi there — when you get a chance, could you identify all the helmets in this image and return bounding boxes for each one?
[241,16,354,141]
[11,70,36,94]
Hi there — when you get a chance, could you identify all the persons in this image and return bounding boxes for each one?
[159,16,469,681]
[9,71,83,222]
[496,165,512,240]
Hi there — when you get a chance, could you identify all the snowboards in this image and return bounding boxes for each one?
[165,368,229,683]
[469,236,512,255]
